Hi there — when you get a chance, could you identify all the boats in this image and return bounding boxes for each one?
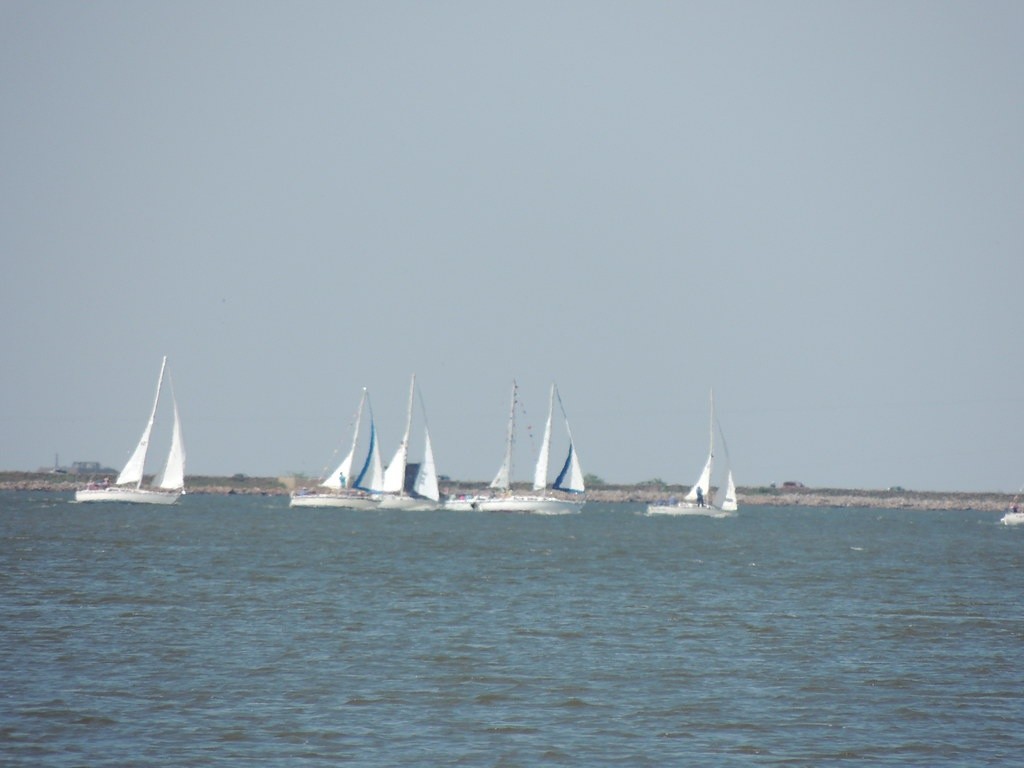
[1000,512,1024,526]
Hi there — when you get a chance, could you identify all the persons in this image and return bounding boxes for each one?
[696,486,705,508]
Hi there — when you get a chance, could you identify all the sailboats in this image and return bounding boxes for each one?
[479,379,587,515]
[445,378,521,511]
[648,387,738,517]
[74,355,187,505]
[373,373,443,511]
[288,386,384,510]
